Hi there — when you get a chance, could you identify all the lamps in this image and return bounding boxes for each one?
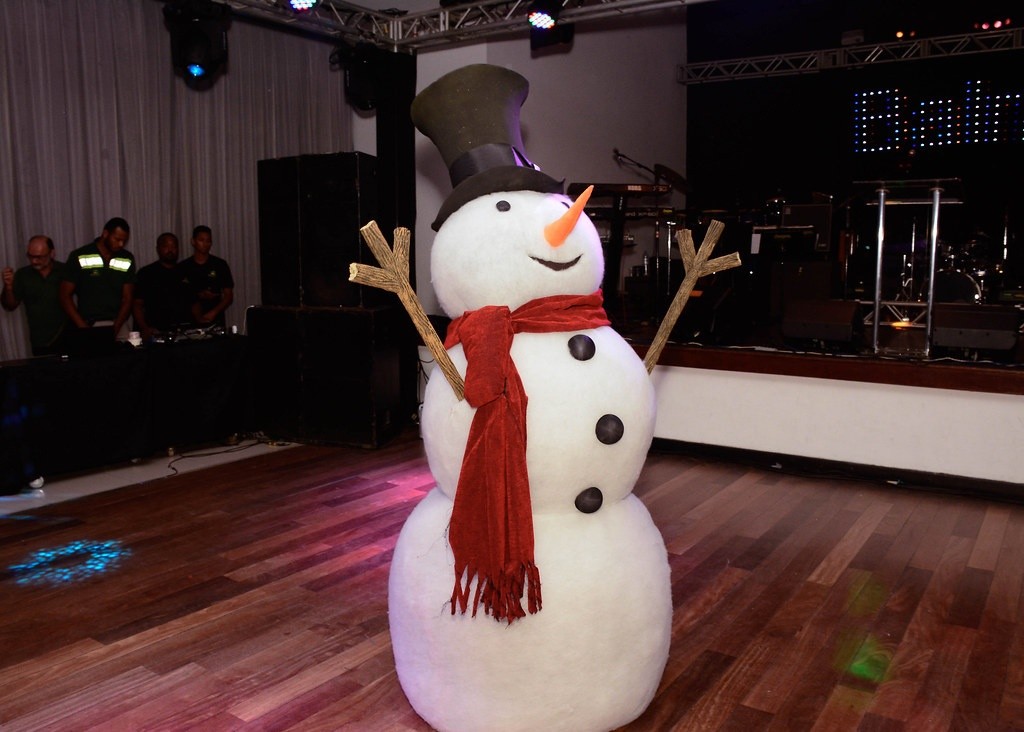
[348,43,387,112]
[161,0,236,92]
[528,0,561,31]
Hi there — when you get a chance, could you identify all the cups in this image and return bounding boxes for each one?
[129,331,141,345]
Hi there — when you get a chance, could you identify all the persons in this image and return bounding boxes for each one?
[0,213,239,361]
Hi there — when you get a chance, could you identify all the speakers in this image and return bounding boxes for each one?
[770,201,867,350]
[931,308,1022,364]
[244,150,408,451]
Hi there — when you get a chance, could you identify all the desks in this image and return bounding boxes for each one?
[0,335,260,496]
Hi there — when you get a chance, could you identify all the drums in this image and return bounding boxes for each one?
[918,232,1016,304]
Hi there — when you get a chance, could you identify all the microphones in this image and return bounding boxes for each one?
[613,148,621,160]
